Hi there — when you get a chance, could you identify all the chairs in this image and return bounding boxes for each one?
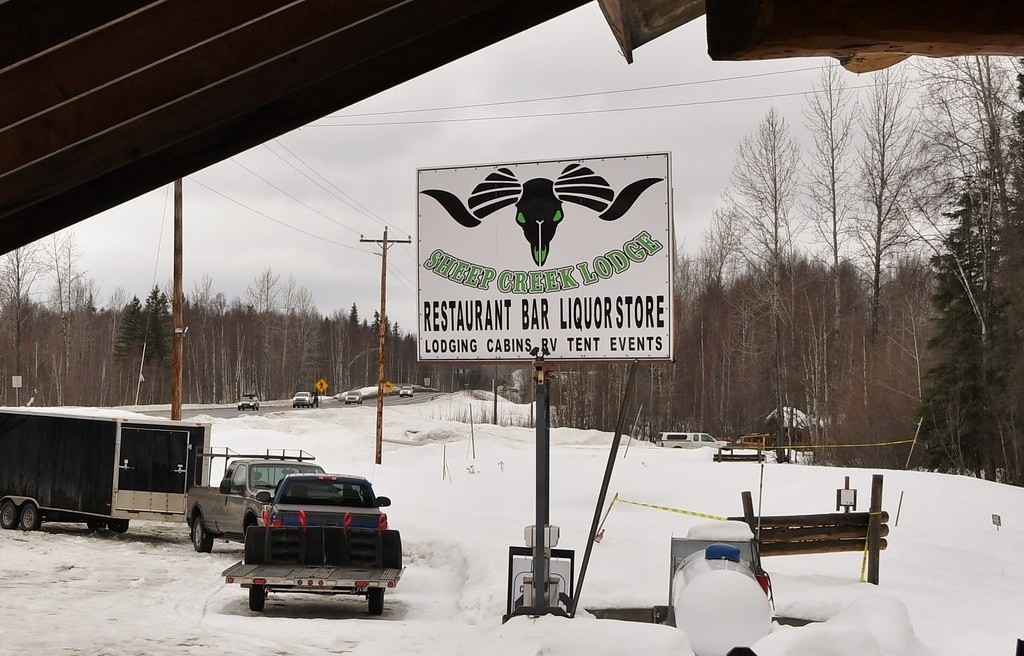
[254,472,267,485]
[291,485,307,498]
[339,490,361,502]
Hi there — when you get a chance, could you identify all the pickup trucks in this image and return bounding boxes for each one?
[221,472,407,615]
[344,390,364,405]
[187,459,327,556]
[292,392,315,408]
[656,431,723,450]
[237,393,260,411]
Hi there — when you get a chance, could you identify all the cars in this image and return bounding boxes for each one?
[399,386,414,397]
